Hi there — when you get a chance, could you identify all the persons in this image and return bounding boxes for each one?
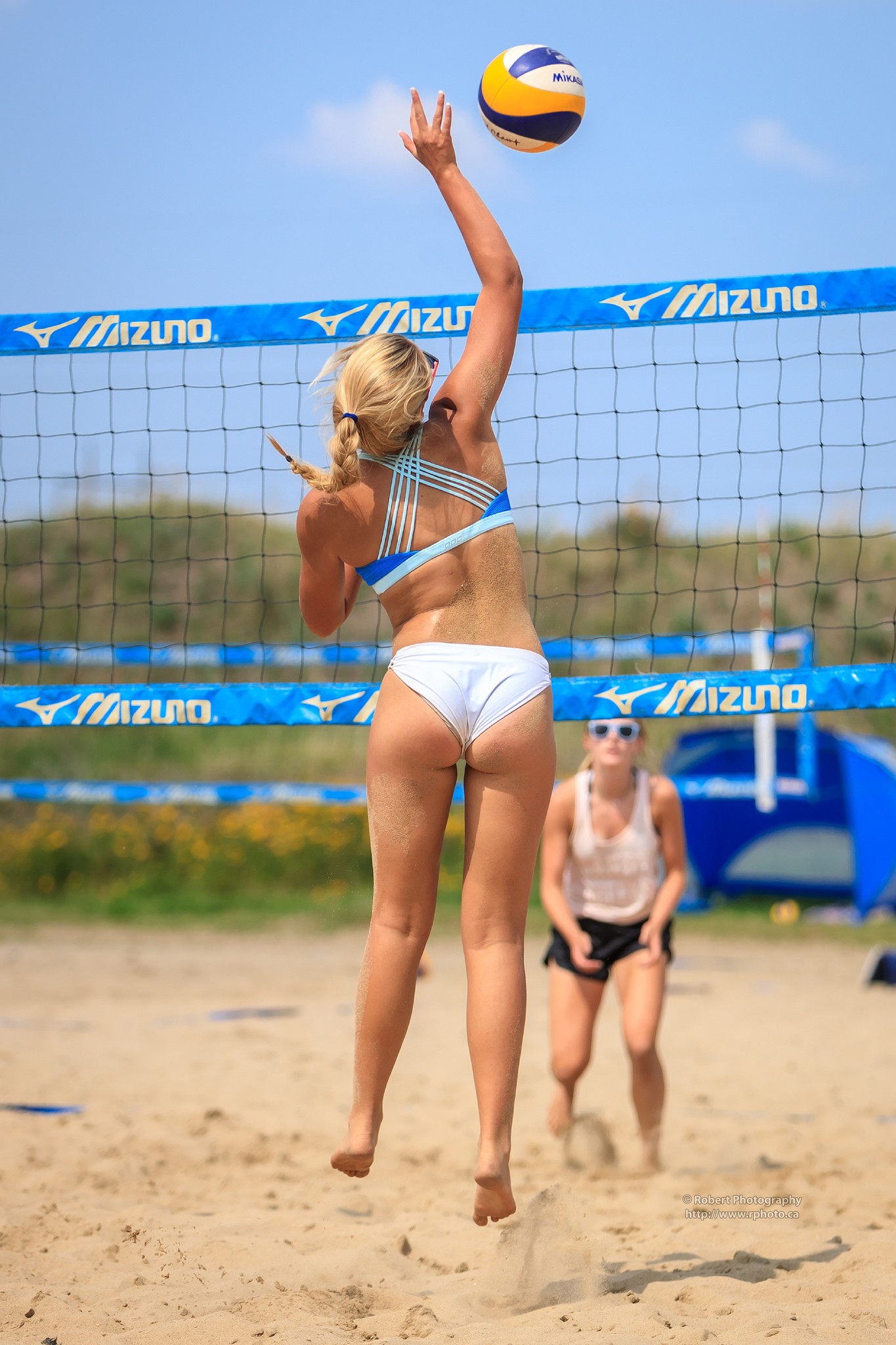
[538,715,688,1183]
[297,86,559,1226]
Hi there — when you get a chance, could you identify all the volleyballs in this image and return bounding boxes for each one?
[769,899,800,925]
[478,44,585,153]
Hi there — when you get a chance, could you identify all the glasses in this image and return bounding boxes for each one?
[407,350,439,401]
[587,722,639,742]
